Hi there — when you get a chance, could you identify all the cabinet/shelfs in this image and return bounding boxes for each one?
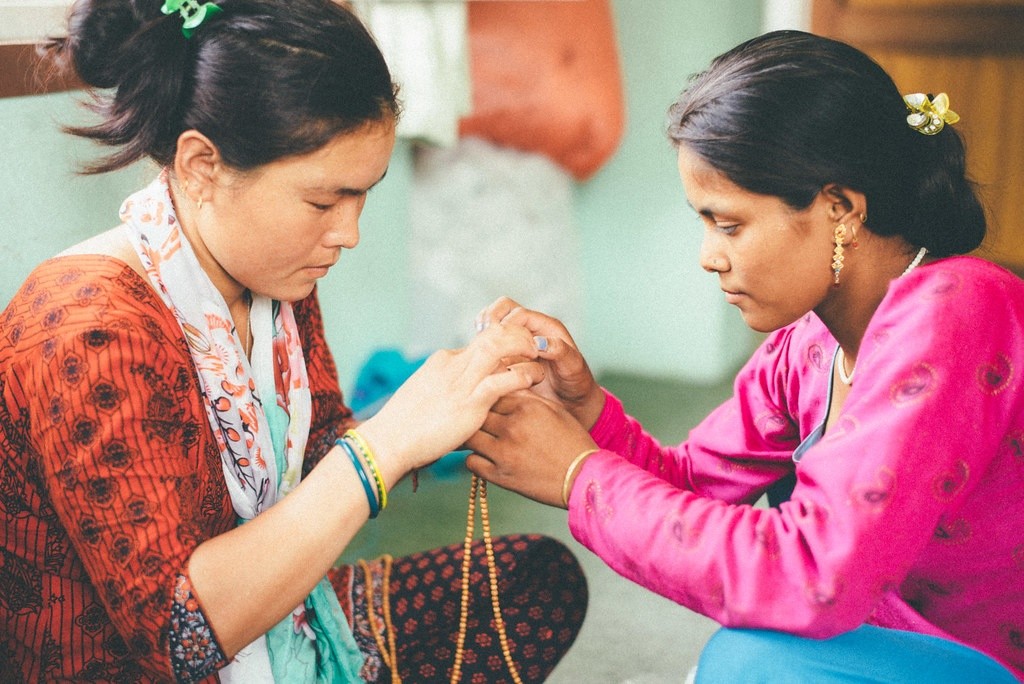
[814,0,1024,280]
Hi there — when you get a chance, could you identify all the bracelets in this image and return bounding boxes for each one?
[334,438,379,520]
[561,448,602,507]
[343,428,387,511]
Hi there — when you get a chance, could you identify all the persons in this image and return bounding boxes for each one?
[3,1,589,684]
[460,29,1023,684]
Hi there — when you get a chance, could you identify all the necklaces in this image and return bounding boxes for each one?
[356,553,403,684]
[447,473,522,684]
[244,289,250,358]
[837,246,927,386]
